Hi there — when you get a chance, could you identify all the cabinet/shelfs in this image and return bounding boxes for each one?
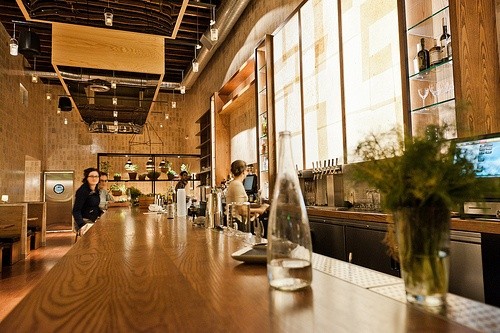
[396,0,500,147]
[210,35,272,204]
[97,153,201,201]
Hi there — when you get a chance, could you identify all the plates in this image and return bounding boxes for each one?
[231,244,291,263]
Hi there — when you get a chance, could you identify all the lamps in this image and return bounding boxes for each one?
[8,0,218,135]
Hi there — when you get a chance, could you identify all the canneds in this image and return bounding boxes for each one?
[167,203,174,219]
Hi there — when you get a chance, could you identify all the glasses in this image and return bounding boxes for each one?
[99,179,108,183]
[87,175,99,178]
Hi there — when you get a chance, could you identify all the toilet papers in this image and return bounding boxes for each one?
[177,189,186,216]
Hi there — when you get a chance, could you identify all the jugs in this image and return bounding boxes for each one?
[205,189,222,229]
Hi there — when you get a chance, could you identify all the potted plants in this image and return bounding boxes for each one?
[100,161,192,180]
[109,184,122,195]
[348,120,498,305]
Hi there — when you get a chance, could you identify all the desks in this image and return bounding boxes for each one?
[0,207,500,333]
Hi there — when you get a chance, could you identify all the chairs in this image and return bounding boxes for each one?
[0,201,48,271]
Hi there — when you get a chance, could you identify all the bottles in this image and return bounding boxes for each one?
[176,188,187,218]
[157,194,162,206]
[367,189,381,208]
[167,201,174,218]
[429,38,442,67]
[440,17,452,62]
[418,38,430,75]
[267,130,313,292]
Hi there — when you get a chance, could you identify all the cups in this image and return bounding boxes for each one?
[227,202,250,238]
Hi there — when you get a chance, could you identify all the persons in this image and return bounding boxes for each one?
[175,171,194,207]
[225,160,267,234]
[72,167,132,237]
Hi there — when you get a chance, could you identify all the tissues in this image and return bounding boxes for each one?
[148,204,162,211]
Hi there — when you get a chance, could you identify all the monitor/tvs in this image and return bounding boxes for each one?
[243,174,258,195]
[450,130,500,202]
